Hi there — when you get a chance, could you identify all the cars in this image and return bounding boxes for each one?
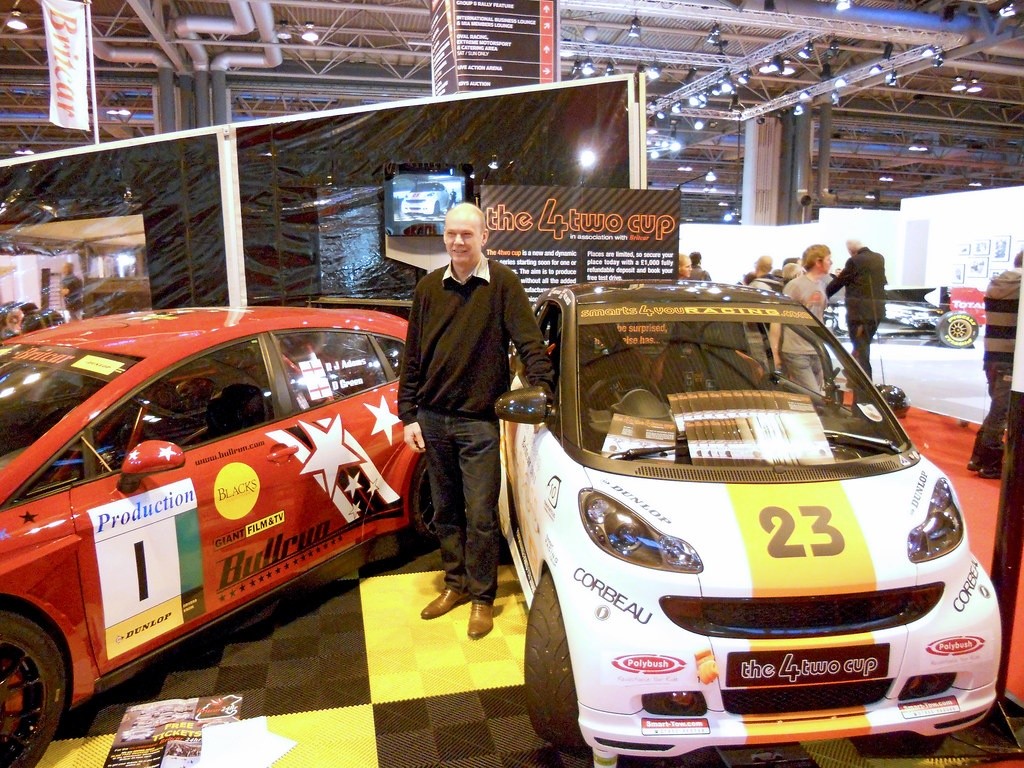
[401,182,450,216]
[0,307,444,768]
[823,283,987,349]
[495,277,1004,759]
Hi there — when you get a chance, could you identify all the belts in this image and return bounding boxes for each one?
[421,404,486,419]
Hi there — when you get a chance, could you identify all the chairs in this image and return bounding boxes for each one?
[206,383,265,438]
[579,323,773,464]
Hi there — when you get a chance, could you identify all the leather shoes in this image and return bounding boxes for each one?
[468,603,494,638]
[421,589,470,620]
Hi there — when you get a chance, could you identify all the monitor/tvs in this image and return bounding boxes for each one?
[382,163,473,236]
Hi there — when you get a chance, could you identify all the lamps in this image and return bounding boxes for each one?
[6,9,27,30]
[14,146,24,154]
[24,145,34,154]
[118,102,130,115]
[302,24,318,41]
[106,100,118,114]
[567,0,1016,223]
[276,19,291,40]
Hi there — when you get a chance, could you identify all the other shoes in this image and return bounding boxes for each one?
[980,465,1001,479]
[967,458,982,470]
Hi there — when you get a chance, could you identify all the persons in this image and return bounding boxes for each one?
[782,244,833,403]
[825,236,889,402]
[966,248,1024,478]
[678,254,692,280]
[689,251,712,280]
[650,322,765,405]
[434,191,452,215]
[397,201,555,639]
[60,262,82,319]
[0,300,65,341]
[737,255,784,374]
